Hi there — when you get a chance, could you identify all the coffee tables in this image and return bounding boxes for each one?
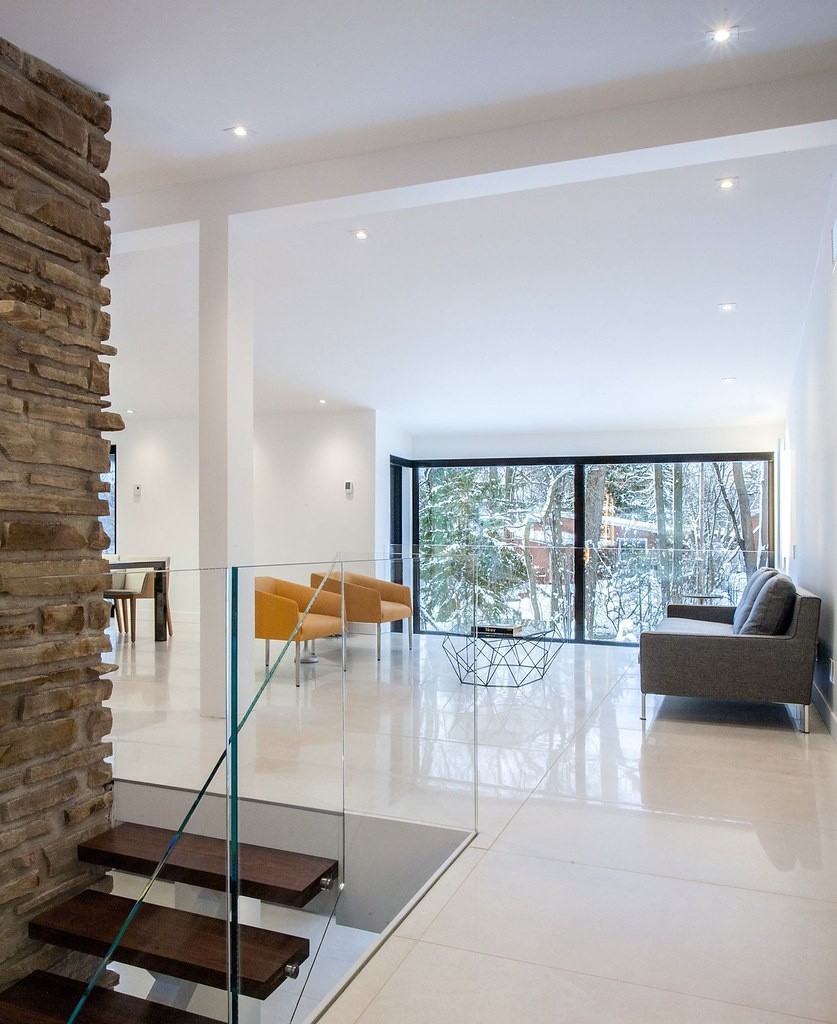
[442,618,566,688]
[437,668,563,750]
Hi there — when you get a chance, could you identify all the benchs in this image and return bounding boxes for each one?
[639,567,823,734]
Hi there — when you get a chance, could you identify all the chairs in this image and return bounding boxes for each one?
[101,553,173,643]
[312,570,414,662]
[252,576,348,687]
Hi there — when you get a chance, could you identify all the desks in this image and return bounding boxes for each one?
[683,595,723,605]
[107,559,167,643]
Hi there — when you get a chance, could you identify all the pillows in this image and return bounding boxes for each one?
[733,567,796,635]
